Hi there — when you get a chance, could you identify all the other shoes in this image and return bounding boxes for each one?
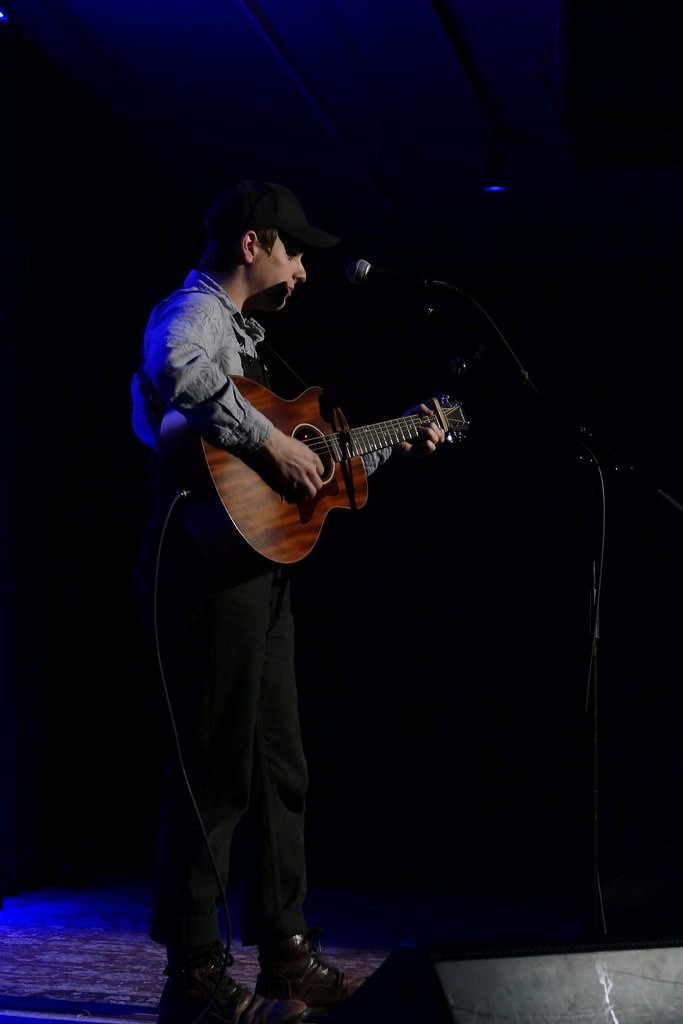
[157,968,311,1023]
[256,956,372,1014]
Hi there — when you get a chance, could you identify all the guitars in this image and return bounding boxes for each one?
[171,377,469,564]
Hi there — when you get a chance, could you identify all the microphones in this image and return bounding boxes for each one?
[347,258,458,294]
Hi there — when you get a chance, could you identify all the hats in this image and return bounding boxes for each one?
[208,181,341,248]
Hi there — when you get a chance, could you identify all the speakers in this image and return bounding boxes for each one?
[326,940,683,1024]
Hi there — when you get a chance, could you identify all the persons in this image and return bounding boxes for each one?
[132,176,446,1024]
